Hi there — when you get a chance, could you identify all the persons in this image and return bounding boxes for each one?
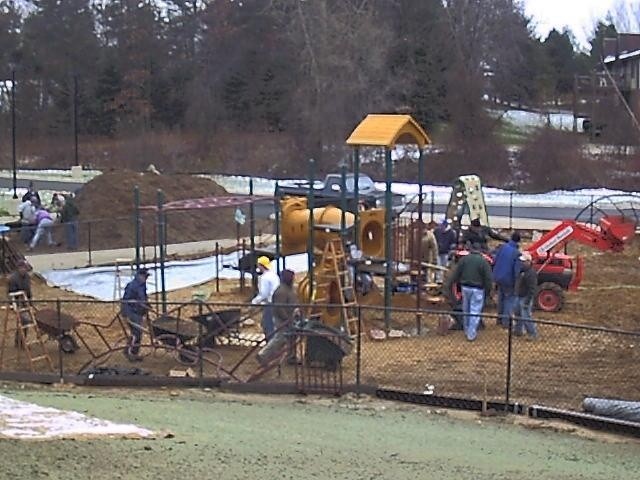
[247,255,282,345]
[433,217,541,342]
[15,180,81,251]
[120,269,156,363]
[255,269,305,369]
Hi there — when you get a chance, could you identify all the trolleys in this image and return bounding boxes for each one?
[294,319,354,371]
[141,303,207,364]
[22,302,79,353]
[191,308,242,345]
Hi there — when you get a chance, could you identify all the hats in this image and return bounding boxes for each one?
[439,220,448,228]
[472,243,480,249]
[512,233,520,242]
[519,254,532,261]
[471,220,480,226]
[258,256,271,271]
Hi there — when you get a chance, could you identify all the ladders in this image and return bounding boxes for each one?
[0,290,55,375]
[303,237,368,345]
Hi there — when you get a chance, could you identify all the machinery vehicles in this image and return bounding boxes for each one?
[444,195,637,315]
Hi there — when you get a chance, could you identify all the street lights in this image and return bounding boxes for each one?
[65,49,84,175]
[9,56,22,201]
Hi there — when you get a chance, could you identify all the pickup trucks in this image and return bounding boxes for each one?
[274,172,407,220]
